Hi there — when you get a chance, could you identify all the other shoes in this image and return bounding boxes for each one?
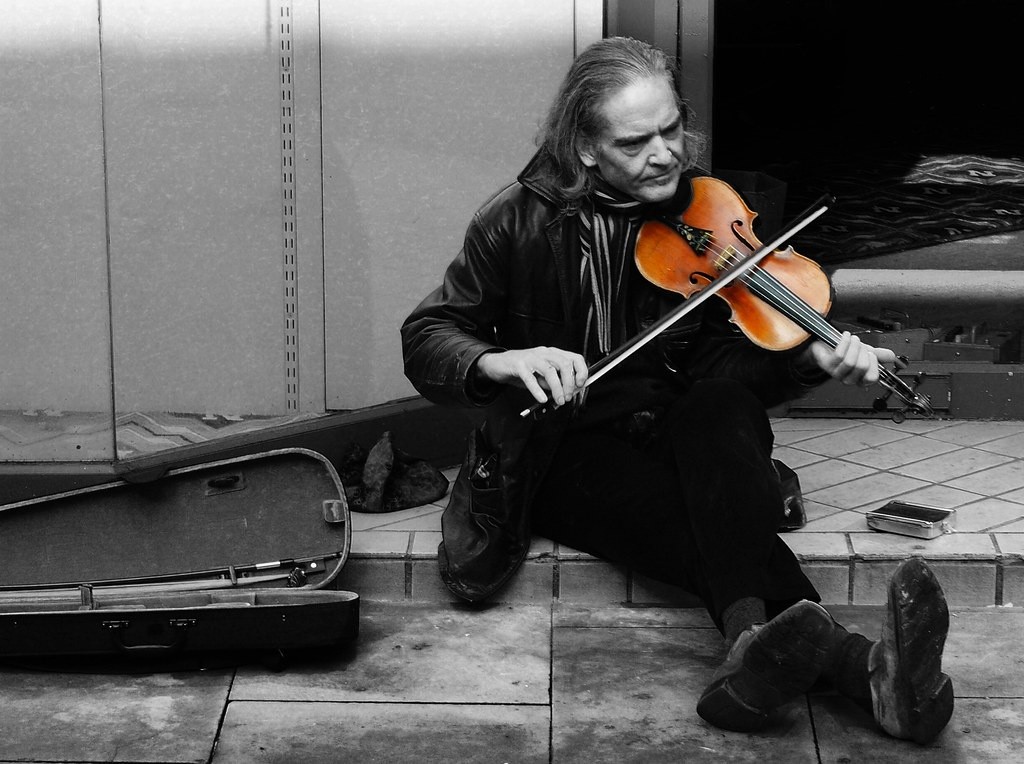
[867,558,955,743]
[696,599,835,735]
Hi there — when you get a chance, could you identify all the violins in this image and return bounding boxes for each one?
[633,175,935,425]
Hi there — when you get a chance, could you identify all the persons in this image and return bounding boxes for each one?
[399,37,954,746]
